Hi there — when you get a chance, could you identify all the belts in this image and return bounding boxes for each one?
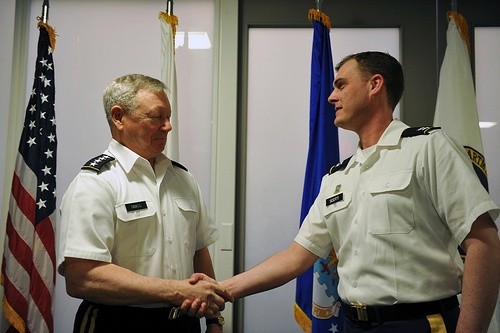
[342,294,460,322]
[95,304,183,321]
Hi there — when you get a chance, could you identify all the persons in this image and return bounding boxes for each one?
[56,73,225,333]
[180,51,500,333]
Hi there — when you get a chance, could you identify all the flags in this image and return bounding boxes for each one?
[0,22,58,333]
[157,11,178,163]
[433,9,500,333]
[294,8,342,333]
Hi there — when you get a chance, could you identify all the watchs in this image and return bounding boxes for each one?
[205,316,225,326]
